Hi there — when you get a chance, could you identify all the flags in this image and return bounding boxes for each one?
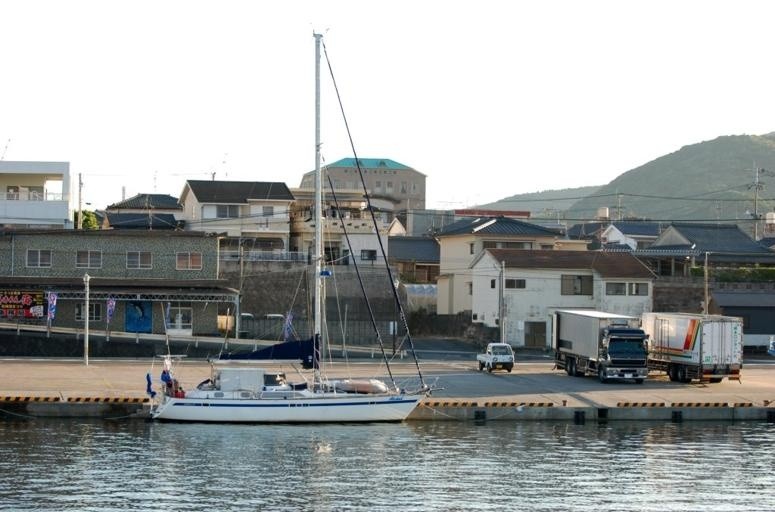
[160,301,170,331]
[103,298,118,325]
[284,311,296,339]
[47,291,59,323]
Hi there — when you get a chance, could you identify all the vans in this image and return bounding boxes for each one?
[240,313,254,318]
[263,314,284,320]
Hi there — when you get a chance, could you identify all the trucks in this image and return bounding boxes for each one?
[630,312,745,386]
[476,342,517,375]
[548,309,650,385]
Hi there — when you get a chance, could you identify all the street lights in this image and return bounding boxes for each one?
[77,201,91,230]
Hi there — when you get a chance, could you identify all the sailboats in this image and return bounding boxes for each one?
[152,31,445,423]
